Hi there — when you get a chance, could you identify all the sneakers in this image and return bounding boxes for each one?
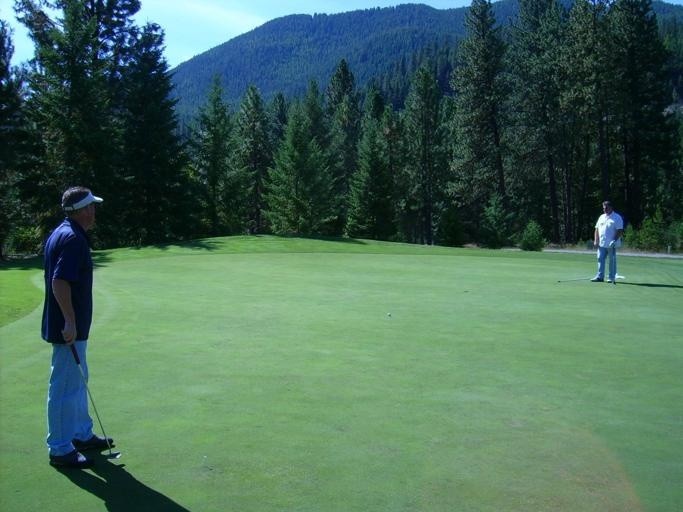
[591,277,604,282]
[72,436,113,452]
[49,449,94,469]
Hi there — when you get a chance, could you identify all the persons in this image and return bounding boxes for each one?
[593,196,624,287]
[39,186,113,468]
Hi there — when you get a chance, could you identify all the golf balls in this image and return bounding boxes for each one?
[388,313,391,316]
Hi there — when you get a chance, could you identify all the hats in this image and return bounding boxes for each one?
[62,191,104,211]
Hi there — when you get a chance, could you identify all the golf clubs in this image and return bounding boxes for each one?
[71,342,121,459]
[612,243,616,285]
